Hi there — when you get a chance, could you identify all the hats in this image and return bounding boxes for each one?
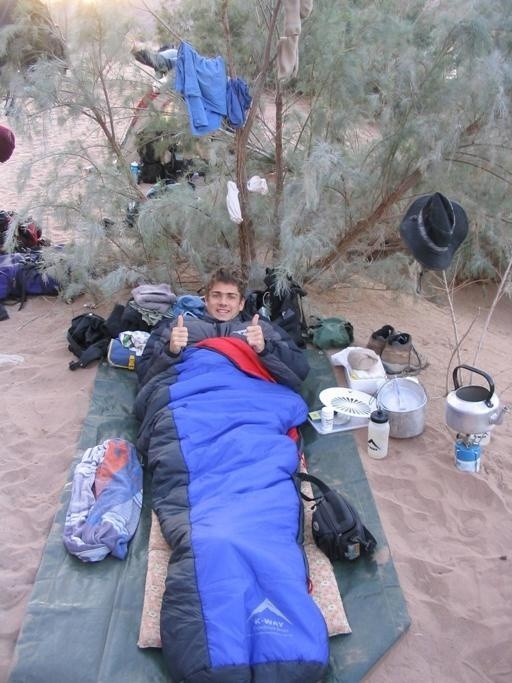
[400,192,468,269]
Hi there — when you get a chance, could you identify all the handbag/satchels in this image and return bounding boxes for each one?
[293,472,376,561]
[107,337,141,370]
[0,252,62,295]
[312,317,353,348]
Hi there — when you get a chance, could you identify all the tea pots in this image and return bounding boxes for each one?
[447,365,508,433]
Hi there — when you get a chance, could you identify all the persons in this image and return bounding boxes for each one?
[133,266,310,382]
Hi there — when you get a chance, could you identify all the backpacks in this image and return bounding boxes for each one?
[264,267,306,345]
[67,313,110,370]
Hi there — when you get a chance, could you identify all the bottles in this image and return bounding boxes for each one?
[369,406,390,459]
[130,160,140,185]
[321,407,334,432]
[128,355,135,371]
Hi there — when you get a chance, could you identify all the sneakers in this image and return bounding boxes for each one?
[367,325,394,356]
[381,332,423,374]
